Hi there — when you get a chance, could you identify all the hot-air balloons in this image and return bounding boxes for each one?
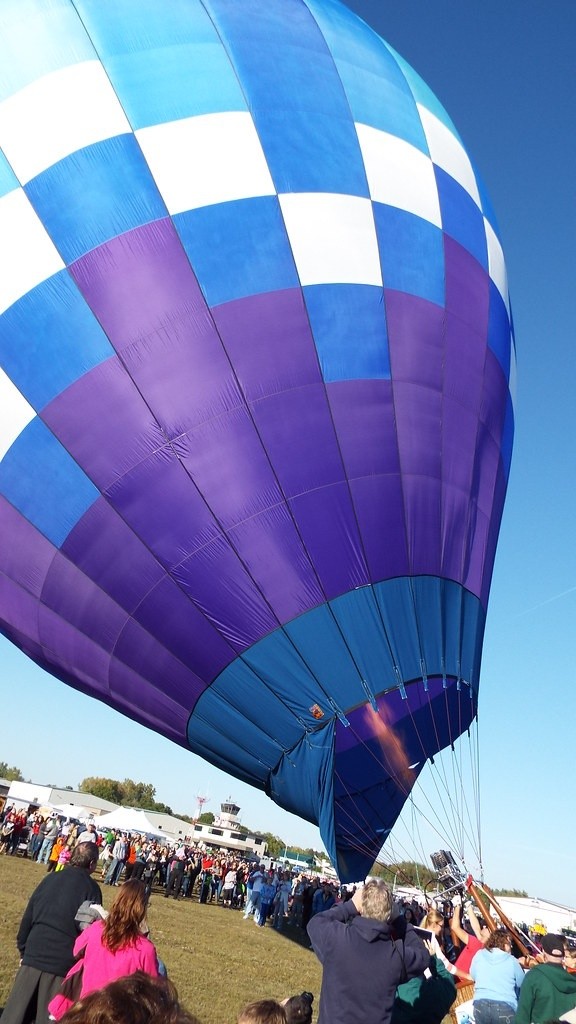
[0,0,545,1024]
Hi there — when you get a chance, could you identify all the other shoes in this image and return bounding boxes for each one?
[255,923,265,928]
[104,883,108,886]
[115,882,119,886]
[36,860,41,863]
[173,897,177,900]
[44,862,47,865]
[242,915,248,919]
[163,895,168,898]
[208,899,212,902]
[254,918,259,923]
[100,875,104,878]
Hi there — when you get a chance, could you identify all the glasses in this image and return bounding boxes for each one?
[147,902,152,908]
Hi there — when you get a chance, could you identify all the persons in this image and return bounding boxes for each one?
[99,828,204,900]
[0,839,102,1023]
[307,881,432,1024]
[237,999,287,1024]
[279,991,314,1024]
[0,797,105,873]
[52,879,182,1024]
[392,893,576,1024]
[198,851,357,945]
[391,939,458,1024]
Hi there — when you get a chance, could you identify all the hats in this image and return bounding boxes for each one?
[540,935,565,957]
[260,864,265,868]
